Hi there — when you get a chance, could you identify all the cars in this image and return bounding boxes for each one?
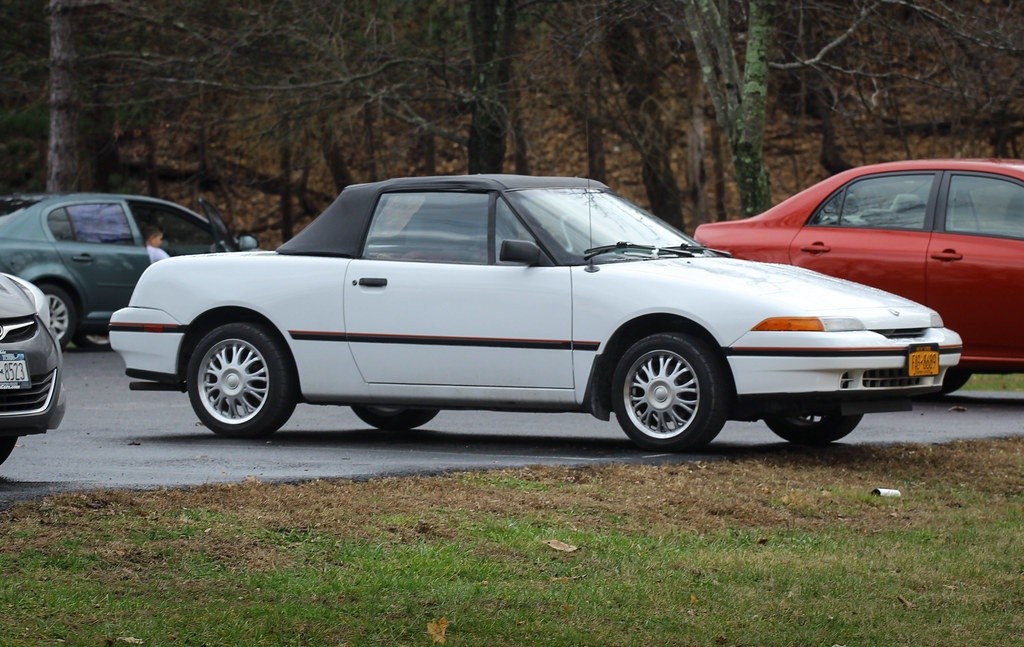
[0,269,65,467]
[690,157,1023,380]
[0,189,260,354]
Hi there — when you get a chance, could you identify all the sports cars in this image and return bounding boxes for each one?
[104,174,966,456]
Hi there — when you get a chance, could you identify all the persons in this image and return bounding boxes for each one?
[140,224,171,264]
